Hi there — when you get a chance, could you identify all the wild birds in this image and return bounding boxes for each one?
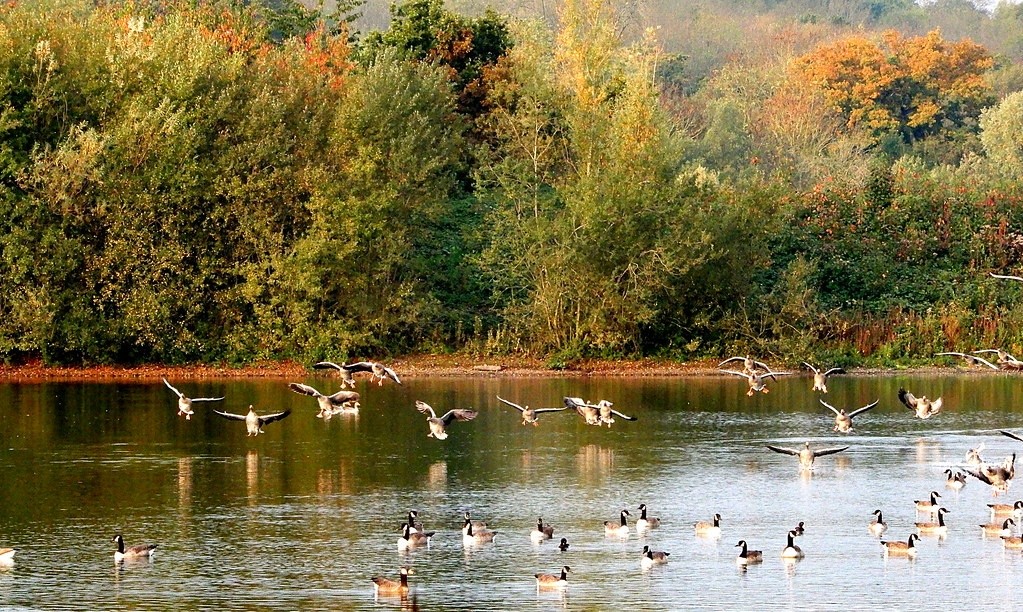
[636,503,660,528]
[880,534,921,554]
[534,566,573,586]
[604,509,632,535]
[163,361,404,437]
[531,518,571,552]
[462,512,498,546]
[717,271,1022,470]
[495,396,637,428]
[111,535,159,558]
[415,400,479,441]
[397,511,436,550]
[734,540,763,563]
[694,513,723,535]
[869,510,888,534]
[913,430,1023,548]
[781,521,805,558]
[370,567,414,592]
[0,547,21,560]
[641,546,670,569]
